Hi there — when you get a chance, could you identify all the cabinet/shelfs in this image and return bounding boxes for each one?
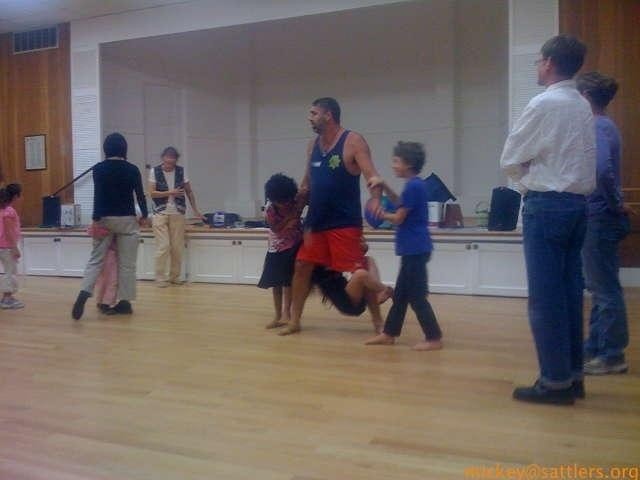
[183,227,269,285]
[135,227,156,280]
[15,226,93,278]
[426,227,529,298]
[363,229,401,288]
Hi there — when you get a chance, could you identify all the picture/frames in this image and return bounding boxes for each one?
[23,134,47,172]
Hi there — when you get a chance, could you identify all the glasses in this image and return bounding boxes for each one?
[532,57,548,66]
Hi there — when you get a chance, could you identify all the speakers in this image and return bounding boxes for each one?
[488,186,521,231]
[43,196,61,227]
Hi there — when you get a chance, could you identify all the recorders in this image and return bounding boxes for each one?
[202,211,238,228]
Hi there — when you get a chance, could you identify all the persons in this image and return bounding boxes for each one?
[91,226,123,312]
[148,147,206,288]
[577,73,630,373]
[71,133,146,324]
[275,97,384,346]
[257,173,303,330]
[500,33,596,407]
[364,142,444,349]
[0,182,24,307]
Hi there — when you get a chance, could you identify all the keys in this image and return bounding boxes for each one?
[310,263,394,317]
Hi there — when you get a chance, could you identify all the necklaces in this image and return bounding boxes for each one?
[317,131,338,158]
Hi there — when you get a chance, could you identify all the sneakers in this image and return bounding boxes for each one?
[583,352,630,375]
[513,381,576,405]
[572,380,586,400]
[0,297,24,308]
